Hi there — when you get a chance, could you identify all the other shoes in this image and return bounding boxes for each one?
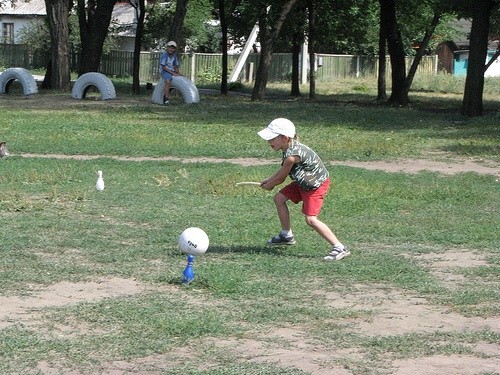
[164,98,169,103]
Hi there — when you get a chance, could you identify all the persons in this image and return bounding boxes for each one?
[257,118,351,262]
[159,40,183,104]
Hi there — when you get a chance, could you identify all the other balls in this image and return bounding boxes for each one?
[179,227,209,255]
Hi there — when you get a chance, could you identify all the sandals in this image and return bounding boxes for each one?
[323,246,350,261]
[267,232,296,244]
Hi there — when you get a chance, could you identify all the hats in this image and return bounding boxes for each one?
[257,118,296,140]
[167,41,177,48]
